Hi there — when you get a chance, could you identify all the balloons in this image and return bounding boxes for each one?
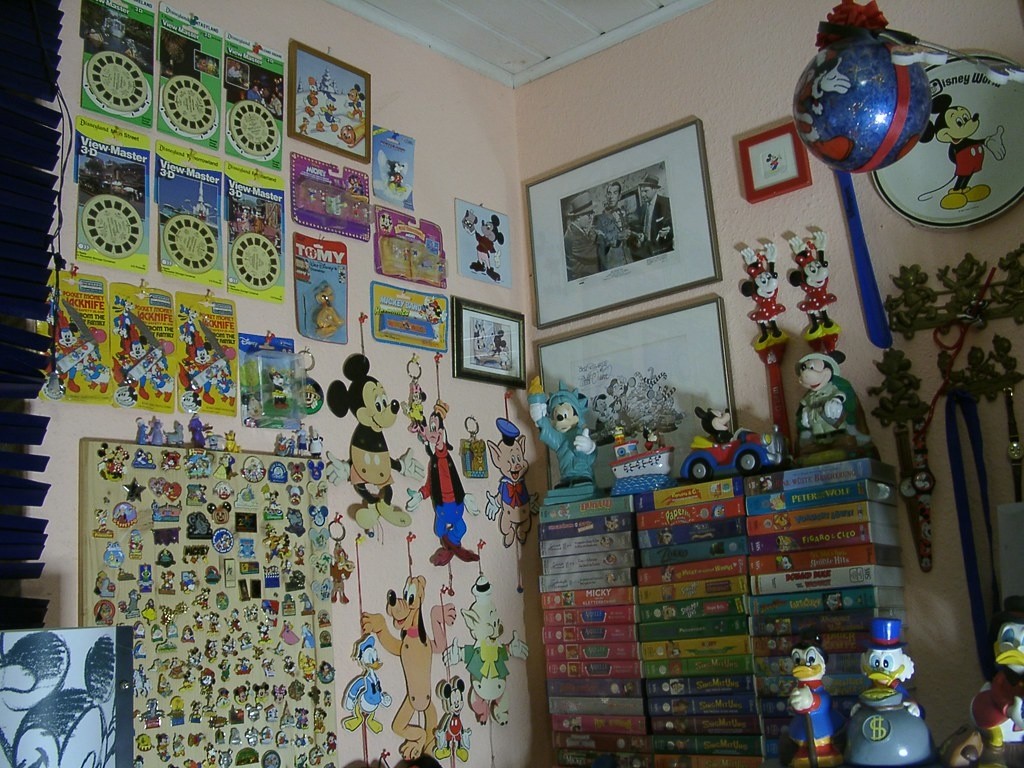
[792,30,936,174]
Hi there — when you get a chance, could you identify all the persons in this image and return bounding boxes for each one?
[563,174,675,282]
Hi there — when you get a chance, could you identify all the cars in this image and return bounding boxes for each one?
[678,405,792,483]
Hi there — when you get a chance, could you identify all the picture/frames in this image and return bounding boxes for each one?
[738,122,812,204]
[450,295,526,391]
[287,37,372,164]
[536,297,738,498]
[525,119,723,331]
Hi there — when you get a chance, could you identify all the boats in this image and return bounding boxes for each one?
[607,421,675,476]
